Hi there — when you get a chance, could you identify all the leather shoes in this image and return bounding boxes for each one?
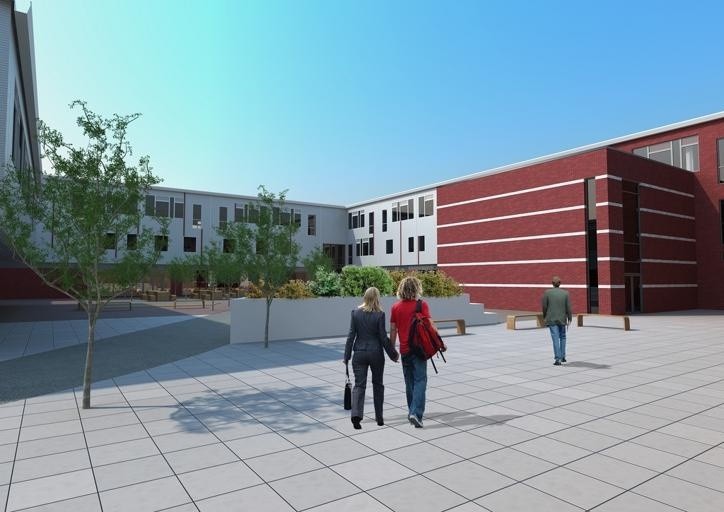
[350,417,383,429]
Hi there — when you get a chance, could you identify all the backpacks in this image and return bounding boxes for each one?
[408,298,443,360]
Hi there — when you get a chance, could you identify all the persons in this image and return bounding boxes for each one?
[343,287,398,429]
[391,276,445,426]
[194,270,203,288]
[541,276,573,365]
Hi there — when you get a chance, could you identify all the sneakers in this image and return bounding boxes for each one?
[553,358,567,365]
[406,412,425,429]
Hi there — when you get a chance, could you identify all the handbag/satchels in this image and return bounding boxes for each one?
[342,382,354,410]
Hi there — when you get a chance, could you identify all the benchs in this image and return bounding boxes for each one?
[431,318,465,334]
[576,313,631,330]
[505,312,545,330]
[78,301,131,312]
